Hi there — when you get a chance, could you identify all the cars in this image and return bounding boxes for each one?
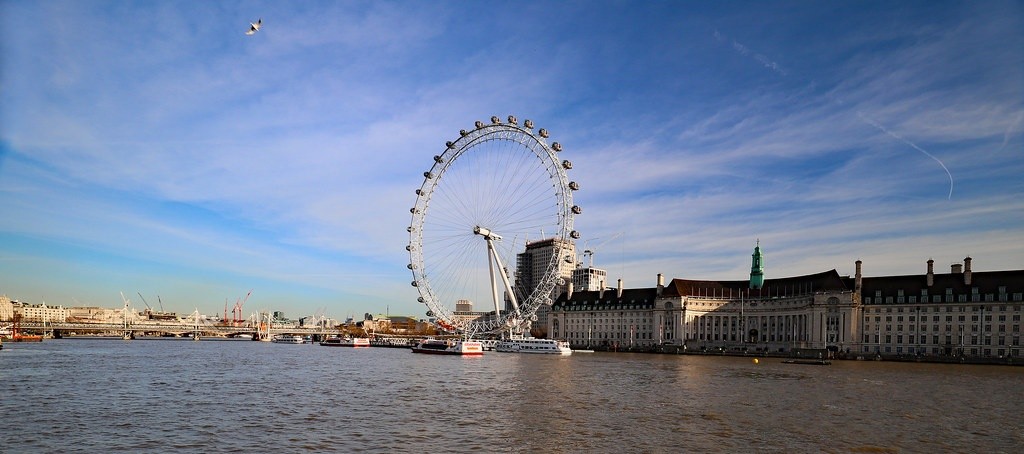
[71,332,76,336]
[234,320,253,328]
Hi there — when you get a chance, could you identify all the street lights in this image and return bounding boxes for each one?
[1008,344,1011,355]
[723,339,726,355]
[941,346,944,355]
[704,338,706,353]
[744,339,747,355]
[766,340,769,356]
[652,339,654,344]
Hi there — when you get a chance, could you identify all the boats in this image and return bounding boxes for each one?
[0,339,3,350]
[320,339,354,347]
[509,335,573,354]
[496,341,521,352]
[13,335,45,343]
[411,339,462,355]
[283,336,300,338]
[780,360,832,365]
[272,338,304,344]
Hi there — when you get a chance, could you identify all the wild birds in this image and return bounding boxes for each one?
[246,18,261,35]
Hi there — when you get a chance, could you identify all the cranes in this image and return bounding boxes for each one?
[232,287,253,325]
[581,229,625,267]
[120,291,130,315]
[502,233,517,290]
[225,299,228,324]
